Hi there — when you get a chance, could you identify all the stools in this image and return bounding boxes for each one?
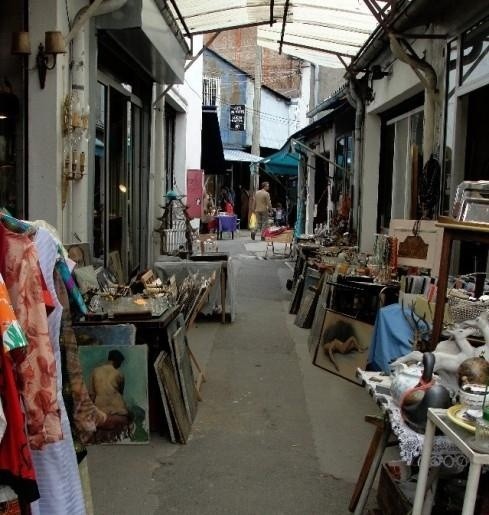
[348,414,392,512]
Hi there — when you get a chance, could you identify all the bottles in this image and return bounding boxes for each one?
[319,223,322,232]
[314,224,319,234]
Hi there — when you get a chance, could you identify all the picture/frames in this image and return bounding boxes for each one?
[73,313,198,446]
[312,308,375,388]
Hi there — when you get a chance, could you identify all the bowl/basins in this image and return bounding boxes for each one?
[460,384,489,408]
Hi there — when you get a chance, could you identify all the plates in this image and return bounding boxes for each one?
[448,404,485,433]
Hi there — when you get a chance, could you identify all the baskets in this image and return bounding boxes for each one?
[447,272,489,323]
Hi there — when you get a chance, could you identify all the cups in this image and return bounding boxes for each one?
[108,297,144,318]
[150,297,168,313]
[475,419,489,448]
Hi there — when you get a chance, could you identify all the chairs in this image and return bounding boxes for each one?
[326,282,368,320]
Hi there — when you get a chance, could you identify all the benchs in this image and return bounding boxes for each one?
[155,261,231,323]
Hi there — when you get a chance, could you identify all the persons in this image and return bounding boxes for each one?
[273,203,283,226]
[323,335,368,372]
[68,245,86,267]
[89,348,127,414]
[250,181,273,240]
[221,187,235,214]
[237,185,250,222]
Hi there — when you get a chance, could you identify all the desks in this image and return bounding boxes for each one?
[353,367,462,515]
[348,281,388,305]
[407,408,488,514]
[214,215,237,240]
[264,230,294,261]
[189,251,229,260]
[430,215,489,353]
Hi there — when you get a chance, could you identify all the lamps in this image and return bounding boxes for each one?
[63,149,87,181]
[371,65,390,79]
[10,31,66,89]
[62,93,90,135]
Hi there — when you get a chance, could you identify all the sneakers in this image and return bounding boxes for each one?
[261,237,265,240]
[251,231,256,240]
